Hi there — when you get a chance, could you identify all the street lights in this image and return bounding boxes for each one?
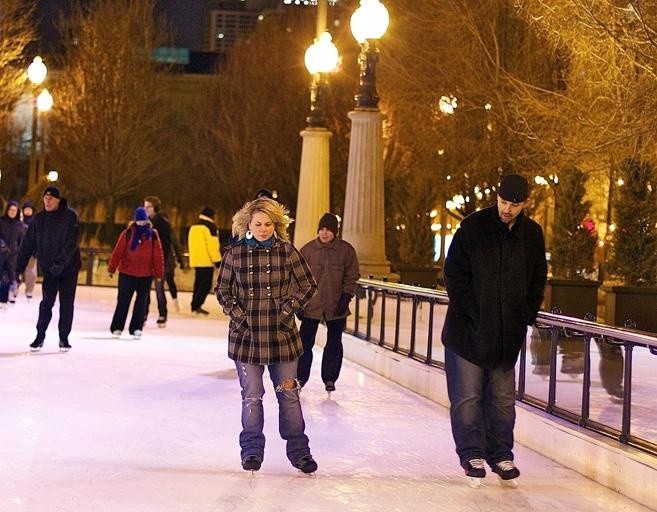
[437,93,457,262]
[340,1,402,283]
[26,55,47,194]
[291,30,340,254]
[35,88,55,179]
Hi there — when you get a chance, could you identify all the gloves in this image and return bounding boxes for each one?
[214,261,222,269]
[177,259,185,270]
[15,270,25,283]
[332,292,354,316]
[48,259,62,273]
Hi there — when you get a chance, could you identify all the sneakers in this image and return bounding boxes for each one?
[112,329,122,335]
[462,457,487,479]
[242,459,262,471]
[490,460,522,480]
[194,306,210,315]
[300,460,318,474]
[325,381,336,392]
[156,313,168,324]
[58,340,73,348]
[134,329,144,336]
[29,337,45,347]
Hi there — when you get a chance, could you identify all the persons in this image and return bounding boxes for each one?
[158,211,184,310]
[188,207,222,314]
[441,175,547,480]
[297,213,359,391]
[108,208,164,337]
[0,201,37,303]
[15,187,80,348]
[214,189,317,473]
[142,197,171,323]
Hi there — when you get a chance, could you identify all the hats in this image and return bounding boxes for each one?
[254,188,273,201]
[316,212,338,238]
[42,186,61,200]
[135,207,148,221]
[497,174,530,203]
[201,207,217,218]
